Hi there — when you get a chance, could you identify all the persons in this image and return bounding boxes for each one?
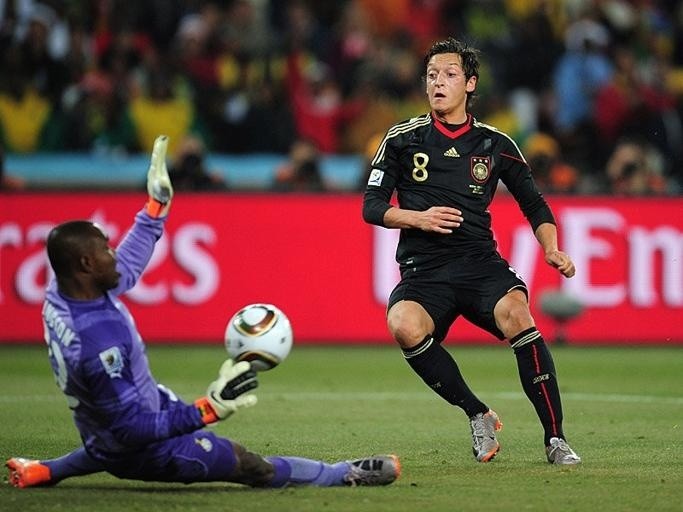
[356,37,581,464]
[0,136,403,489]
[1,0,682,196]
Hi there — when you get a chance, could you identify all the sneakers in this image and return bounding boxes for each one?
[344,452,401,487]
[467,406,503,463]
[6,455,51,489]
[544,436,583,466]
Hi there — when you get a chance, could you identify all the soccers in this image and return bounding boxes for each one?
[226,303,293,373]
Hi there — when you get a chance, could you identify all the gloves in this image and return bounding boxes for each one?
[145,132,174,220]
[192,358,260,426]
